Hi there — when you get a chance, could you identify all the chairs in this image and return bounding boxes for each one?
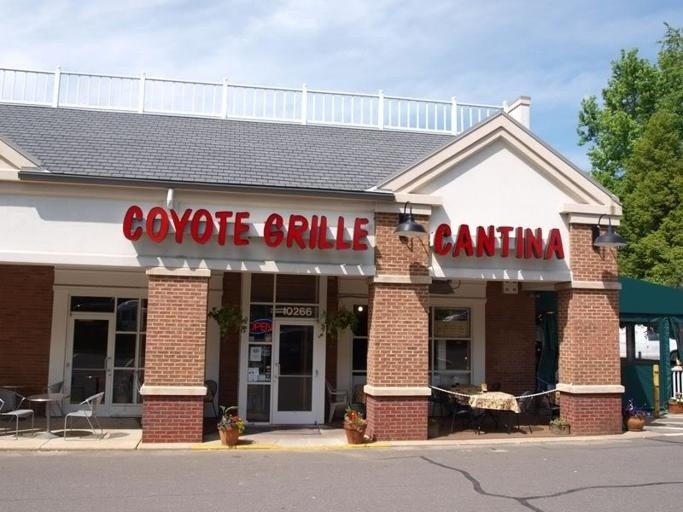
[204,380,216,417]
[0,388,34,439]
[64,392,104,439]
[430,382,535,434]
[325,378,348,423]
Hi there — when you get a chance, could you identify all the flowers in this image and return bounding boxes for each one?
[216,405,245,434]
[343,407,366,431]
[623,398,647,417]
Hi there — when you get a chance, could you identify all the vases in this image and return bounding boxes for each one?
[344,423,362,445]
[217,429,241,445]
[623,415,647,432]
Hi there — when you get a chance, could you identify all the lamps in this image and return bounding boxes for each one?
[393,201,427,244]
[591,213,628,252]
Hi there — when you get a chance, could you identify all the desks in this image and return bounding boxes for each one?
[27,393,70,431]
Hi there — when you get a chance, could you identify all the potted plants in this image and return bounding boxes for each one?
[549,416,570,437]
[207,305,246,340]
[321,304,360,340]
